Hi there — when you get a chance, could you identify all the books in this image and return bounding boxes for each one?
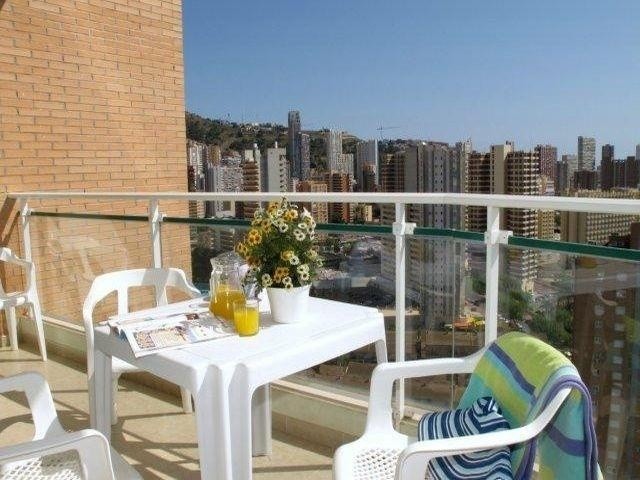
[106,307,237,361]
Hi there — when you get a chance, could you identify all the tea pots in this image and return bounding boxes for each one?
[209,249,247,320]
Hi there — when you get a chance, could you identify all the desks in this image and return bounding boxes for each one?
[91,292,387,480]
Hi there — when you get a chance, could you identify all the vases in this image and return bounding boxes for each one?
[266,284,312,324]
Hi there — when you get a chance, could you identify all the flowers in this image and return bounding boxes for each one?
[238,199,323,289]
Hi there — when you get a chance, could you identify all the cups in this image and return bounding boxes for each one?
[232,297,262,337]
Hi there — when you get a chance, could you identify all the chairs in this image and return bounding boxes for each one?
[0,247,48,363]
[80,263,202,445]
[332,335,573,480]
[3,368,147,479]
